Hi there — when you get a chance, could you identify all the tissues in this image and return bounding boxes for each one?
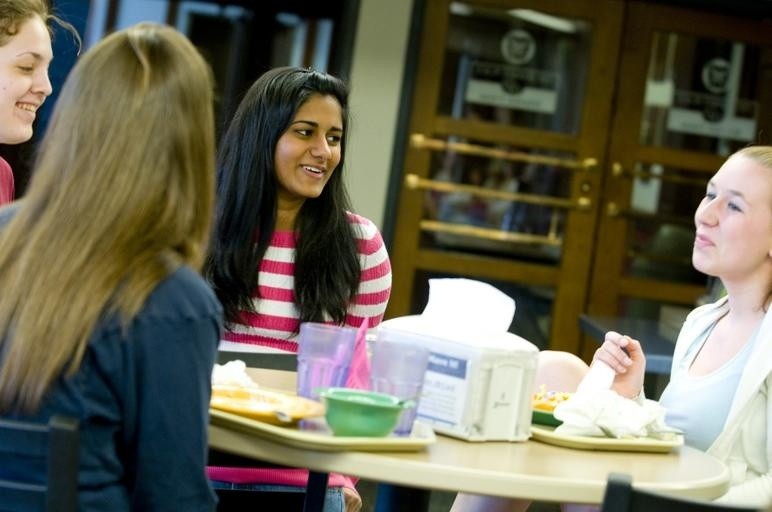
[371,276,539,444]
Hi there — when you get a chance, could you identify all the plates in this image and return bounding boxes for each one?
[202,386,328,428]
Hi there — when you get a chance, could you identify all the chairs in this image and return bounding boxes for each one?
[600,474,760,512]
[0,416,80,512]
[206,351,331,512]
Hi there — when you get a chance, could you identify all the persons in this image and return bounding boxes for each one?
[0,1,84,205]
[0,18,227,509]
[447,147,771,512]
[421,150,704,283]
[202,62,395,510]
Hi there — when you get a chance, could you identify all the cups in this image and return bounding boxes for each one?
[368,341,429,436]
[296,319,357,432]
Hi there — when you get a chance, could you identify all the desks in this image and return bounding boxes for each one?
[208,368,728,506]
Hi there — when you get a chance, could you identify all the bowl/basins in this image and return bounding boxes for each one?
[316,388,416,439]
